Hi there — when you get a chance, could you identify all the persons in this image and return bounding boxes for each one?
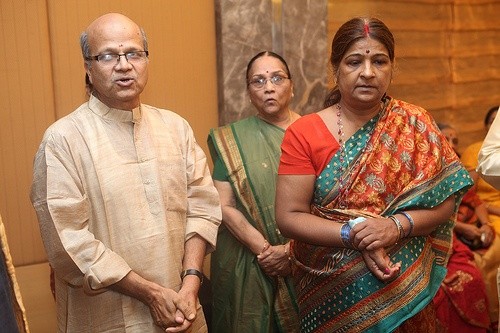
[29,13,223,333]
[205,50,304,333]
[275,17,500,333]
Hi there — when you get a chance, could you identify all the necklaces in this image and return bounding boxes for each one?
[335,98,384,225]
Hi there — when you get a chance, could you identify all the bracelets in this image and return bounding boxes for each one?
[389,215,406,239]
[180,269,206,286]
[338,217,368,249]
[396,210,414,238]
[388,216,401,243]
[260,239,271,254]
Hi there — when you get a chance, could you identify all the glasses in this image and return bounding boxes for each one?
[247,75,289,88]
[85,50,148,64]
